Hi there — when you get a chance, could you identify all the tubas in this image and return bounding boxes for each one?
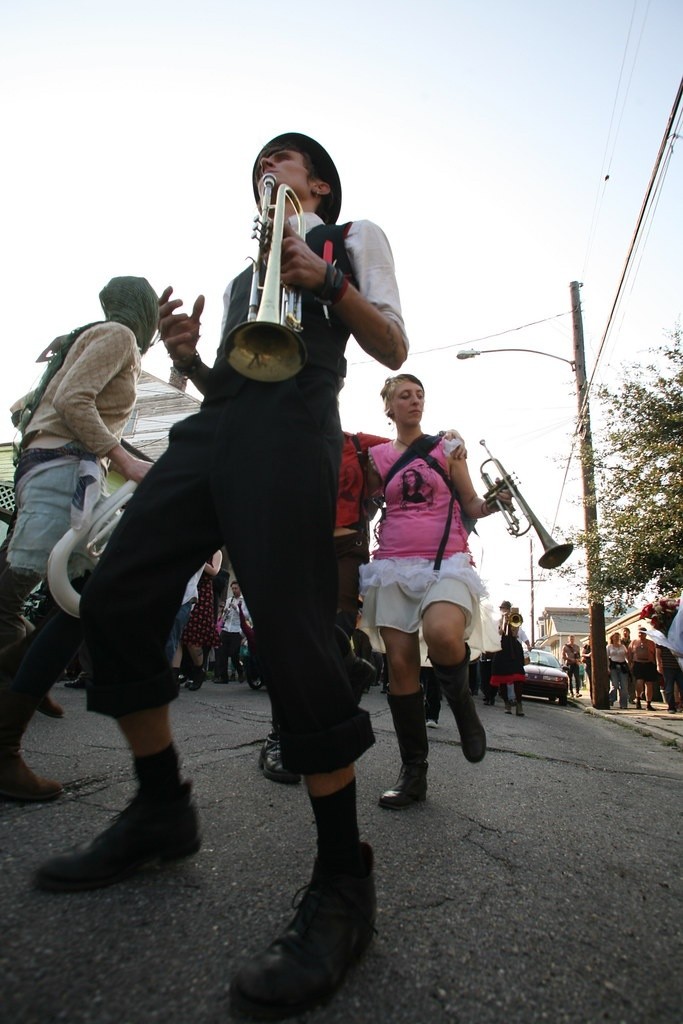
[46,479,141,619]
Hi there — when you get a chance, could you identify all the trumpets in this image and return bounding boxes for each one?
[480,440,573,569]
[224,174,308,383]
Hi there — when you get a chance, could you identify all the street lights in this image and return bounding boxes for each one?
[457,348,611,712]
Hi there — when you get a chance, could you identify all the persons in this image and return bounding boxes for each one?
[32,131,410,1021]
[0,276,180,805]
[362,374,519,809]
[561,635,582,698]
[56,540,531,785]
[255,430,467,785]
[605,611,683,714]
[581,636,593,702]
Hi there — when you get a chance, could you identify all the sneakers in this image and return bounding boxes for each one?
[33,782,204,892]
[231,841,377,1019]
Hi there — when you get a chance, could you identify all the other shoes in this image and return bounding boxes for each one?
[246,649,263,690]
[609,698,656,711]
[669,709,675,713]
[426,719,438,729]
[570,692,582,697]
[170,669,181,684]
[229,673,236,681]
[189,668,204,690]
[349,657,376,704]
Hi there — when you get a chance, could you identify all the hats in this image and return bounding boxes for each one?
[252,132,342,224]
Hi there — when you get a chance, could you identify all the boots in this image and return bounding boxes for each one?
[504,702,512,713]
[516,704,525,716]
[220,664,229,684]
[0,689,65,801]
[428,642,486,763]
[379,682,429,809]
[259,723,302,783]
[238,672,243,682]
[214,668,222,682]
[0,602,65,717]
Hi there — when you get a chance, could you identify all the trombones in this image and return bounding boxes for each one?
[499,610,524,638]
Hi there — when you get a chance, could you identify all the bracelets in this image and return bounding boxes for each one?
[481,501,490,517]
[173,350,204,383]
[309,261,350,308]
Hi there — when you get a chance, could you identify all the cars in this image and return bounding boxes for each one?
[521,648,570,709]
